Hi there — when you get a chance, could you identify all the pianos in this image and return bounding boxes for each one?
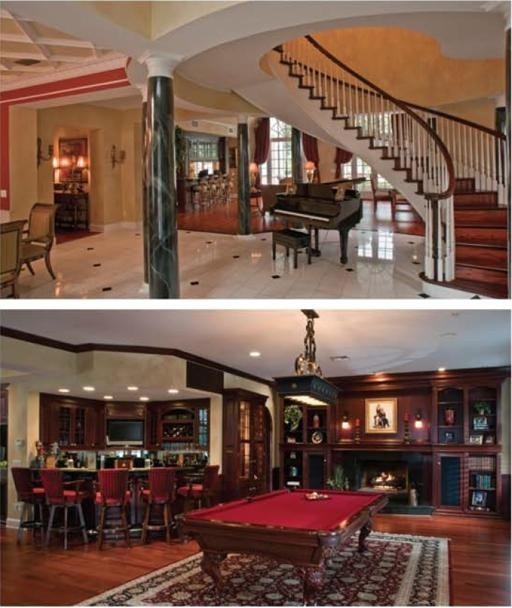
[269,178,366,264]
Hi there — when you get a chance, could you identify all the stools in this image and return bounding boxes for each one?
[271,230,313,269]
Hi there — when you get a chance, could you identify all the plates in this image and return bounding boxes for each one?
[311,431,324,444]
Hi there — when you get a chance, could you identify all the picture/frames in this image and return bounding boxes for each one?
[363,395,401,436]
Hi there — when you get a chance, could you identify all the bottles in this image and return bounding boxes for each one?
[312,414,320,427]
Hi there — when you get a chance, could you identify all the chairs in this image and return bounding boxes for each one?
[20,202,58,285]
[368,176,397,215]
[11,458,219,551]
[0,219,28,299]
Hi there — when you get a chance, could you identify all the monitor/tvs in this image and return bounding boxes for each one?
[106,421,144,448]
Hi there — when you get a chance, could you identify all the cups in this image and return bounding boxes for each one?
[144,458,154,469]
[68,459,74,468]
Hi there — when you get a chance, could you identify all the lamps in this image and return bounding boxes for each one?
[281,309,342,408]
[36,137,54,168]
[111,144,127,170]
[248,159,260,193]
[301,160,318,183]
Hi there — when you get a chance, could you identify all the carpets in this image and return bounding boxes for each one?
[74,526,452,606]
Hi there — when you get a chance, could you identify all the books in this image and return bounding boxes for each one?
[468,456,496,489]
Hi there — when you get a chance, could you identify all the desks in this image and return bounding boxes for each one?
[175,483,388,607]
[55,189,87,231]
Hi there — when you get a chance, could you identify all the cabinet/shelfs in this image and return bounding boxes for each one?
[38,392,105,451]
[278,446,333,490]
[433,372,503,445]
[278,381,334,444]
[145,396,211,451]
[223,386,270,499]
[433,447,503,521]
